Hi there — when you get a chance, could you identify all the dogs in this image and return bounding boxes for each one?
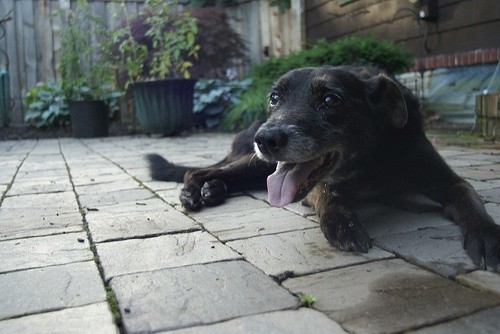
[142,62,500,273]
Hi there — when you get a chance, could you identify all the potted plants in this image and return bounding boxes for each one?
[112,0,200,135]
[48,0,121,137]
